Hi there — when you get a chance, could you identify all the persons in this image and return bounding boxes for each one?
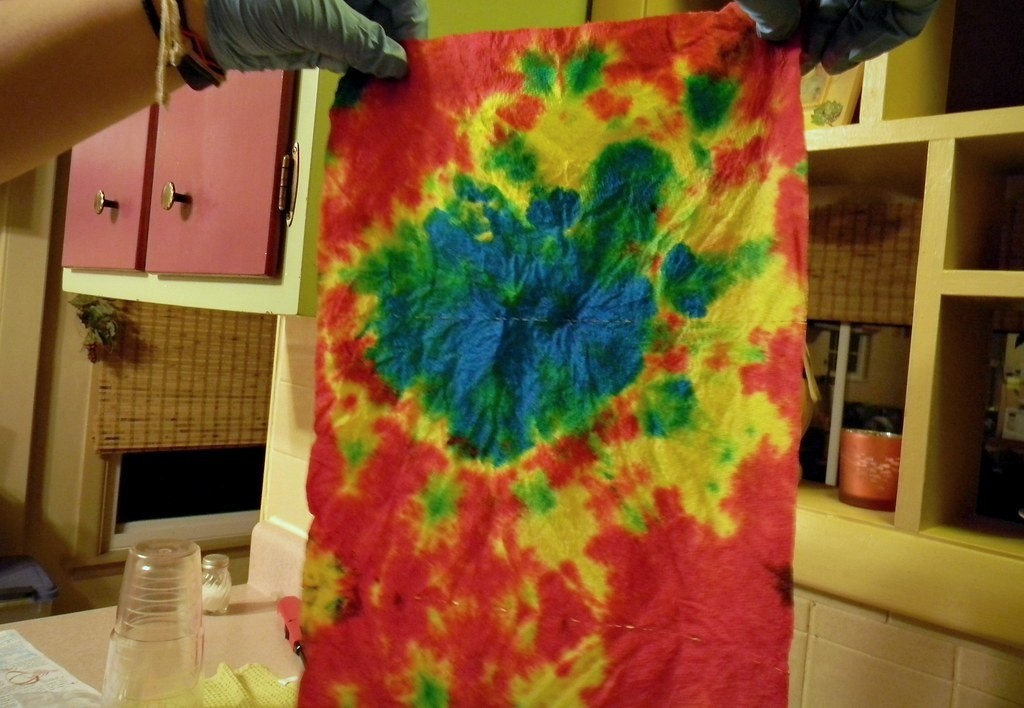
[0,1,939,184]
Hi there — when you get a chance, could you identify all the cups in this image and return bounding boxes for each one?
[840,429,902,512]
[103,539,204,708]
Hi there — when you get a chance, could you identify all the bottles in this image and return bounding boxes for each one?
[202,554,231,613]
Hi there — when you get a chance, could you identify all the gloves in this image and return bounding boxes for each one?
[203,0,429,79]
[735,0,936,75]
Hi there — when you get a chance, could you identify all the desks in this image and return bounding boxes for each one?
[0,584,304,708]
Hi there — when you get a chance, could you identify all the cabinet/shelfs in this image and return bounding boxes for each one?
[794,0,1024,647]
[61,67,344,318]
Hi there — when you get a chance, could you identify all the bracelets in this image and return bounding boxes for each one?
[141,0,227,91]
